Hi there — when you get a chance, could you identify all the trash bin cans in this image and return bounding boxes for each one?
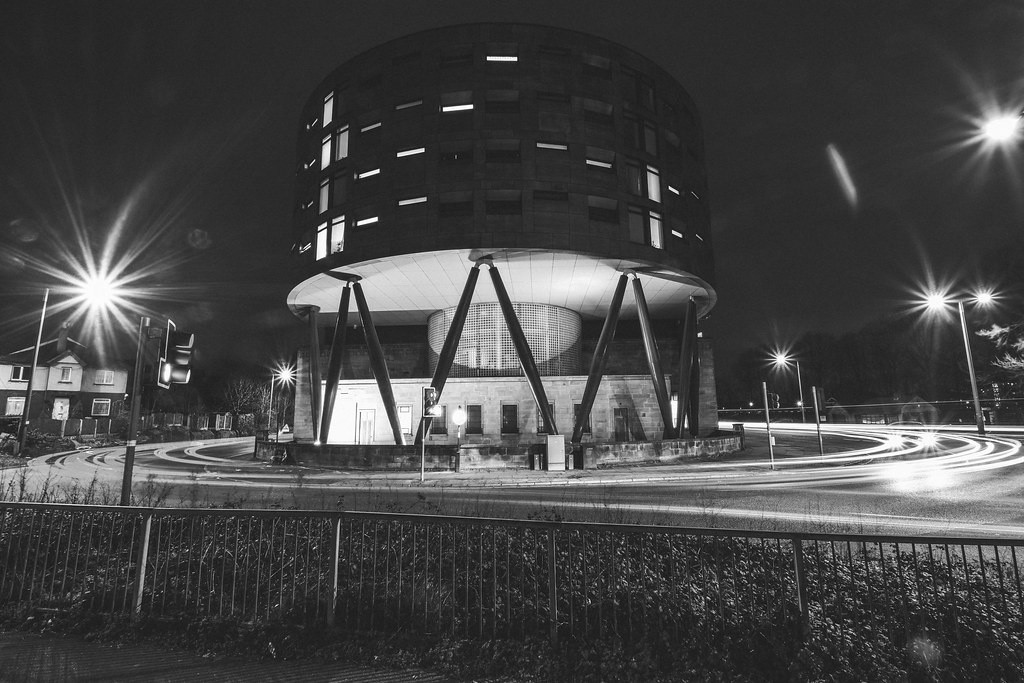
[527,443,547,471]
[569,444,584,470]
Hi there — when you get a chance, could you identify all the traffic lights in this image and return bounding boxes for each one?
[423,387,437,417]
[156,319,195,390]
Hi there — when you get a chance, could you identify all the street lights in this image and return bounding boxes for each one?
[268,372,289,428]
[779,357,805,423]
[18,287,105,457]
[453,405,466,472]
[931,296,988,436]
